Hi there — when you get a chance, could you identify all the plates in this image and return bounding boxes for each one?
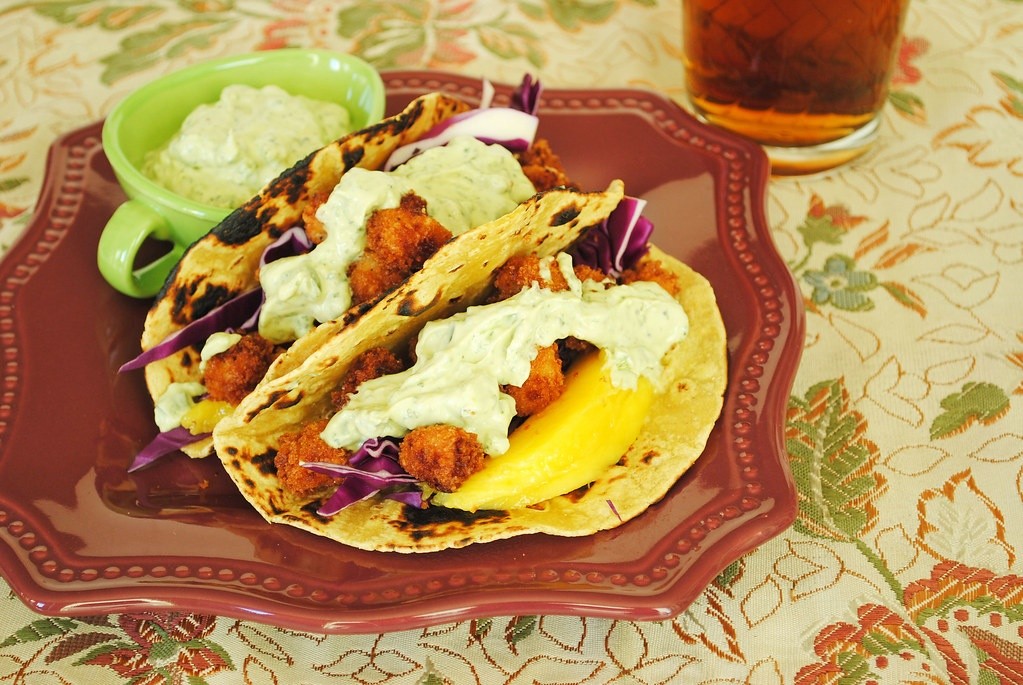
[0,71,807,634]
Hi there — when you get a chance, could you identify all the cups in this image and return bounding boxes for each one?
[683,0,912,180]
[97,49,386,298]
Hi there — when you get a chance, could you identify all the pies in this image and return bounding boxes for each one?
[118,83,729,554]
